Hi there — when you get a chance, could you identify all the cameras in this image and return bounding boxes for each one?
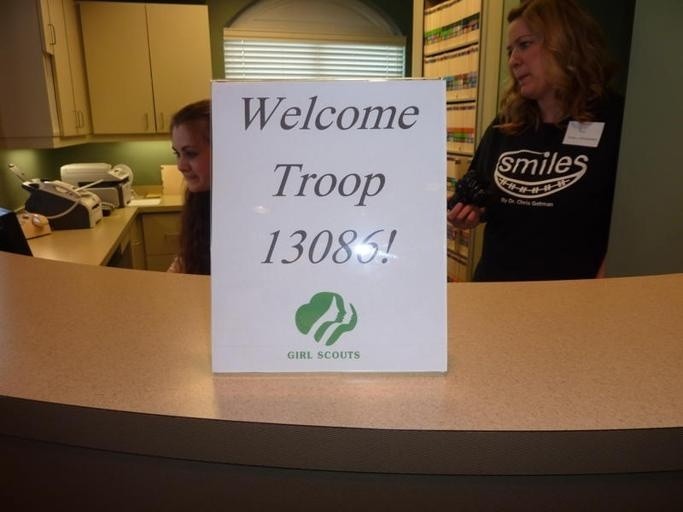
[448,172,488,211]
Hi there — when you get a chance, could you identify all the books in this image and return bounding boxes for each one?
[423,0,481,283]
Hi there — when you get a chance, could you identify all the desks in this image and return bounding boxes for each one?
[26,205,139,266]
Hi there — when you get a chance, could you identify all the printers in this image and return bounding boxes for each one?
[59,162,133,209]
[8,163,104,229]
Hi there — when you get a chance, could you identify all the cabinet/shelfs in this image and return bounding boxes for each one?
[140,211,183,272]
[0,1,214,149]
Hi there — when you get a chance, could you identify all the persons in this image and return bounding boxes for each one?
[446,1,623,282]
[165,98,211,274]
